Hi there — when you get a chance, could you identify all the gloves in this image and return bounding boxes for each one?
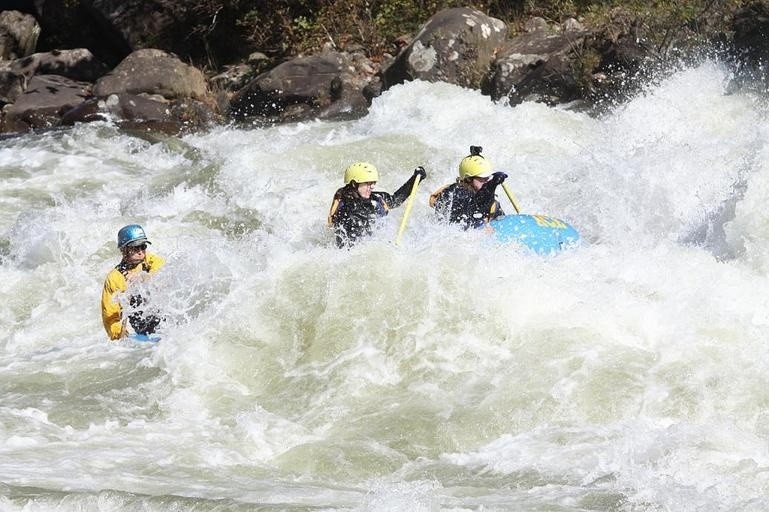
[492,172,508,182]
[413,166,427,180]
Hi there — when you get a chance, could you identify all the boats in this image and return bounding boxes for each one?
[126,215,579,343]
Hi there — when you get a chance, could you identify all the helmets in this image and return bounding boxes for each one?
[458,154,491,181]
[117,224,147,249]
[344,163,379,186]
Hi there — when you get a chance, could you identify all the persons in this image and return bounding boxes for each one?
[330,162,427,253]
[100,224,170,342]
[437,155,508,234]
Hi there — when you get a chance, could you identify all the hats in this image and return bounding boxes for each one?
[127,239,152,248]
[476,170,499,179]
